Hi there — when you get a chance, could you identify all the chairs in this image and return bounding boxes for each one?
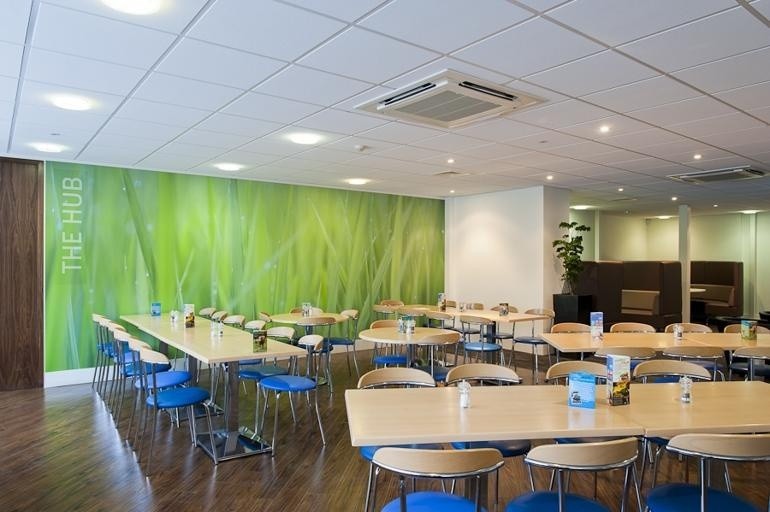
[504,436,642,511]
[361,446,504,512]
[198,306,266,406]
[633,357,720,484]
[359,298,562,384]
[126,336,193,451]
[543,360,637,497]
[445,364,538,497]
[135,346,218,478]
[646,432,769,512]
[256,305,359,375]
[542,319,770,380]
[257,333,326,457]
[233,325,299,437]
[89,313,172,428]
[357,369,449,507]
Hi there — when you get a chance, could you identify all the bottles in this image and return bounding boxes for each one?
[304,305,313,317]
[457,378,472,407]
[679,374,692,404]
[210,316,225,336]
[170,308,179,323]
[406,321,412,336]
[673,324,683,339]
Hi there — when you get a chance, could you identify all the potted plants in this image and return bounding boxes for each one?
[552,219,595,325]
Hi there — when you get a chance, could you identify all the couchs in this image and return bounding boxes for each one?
[568,254,746,324]
